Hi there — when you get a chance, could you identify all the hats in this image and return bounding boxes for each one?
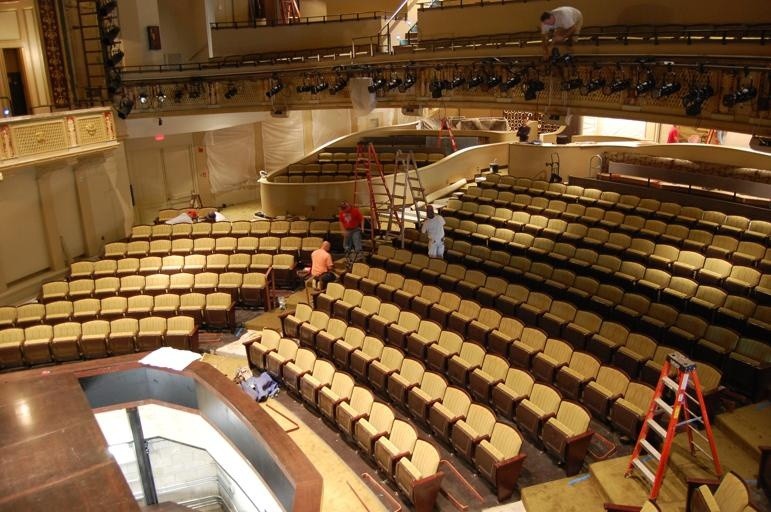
[337,199,350,207]
[426,205,433,211]
[208,211,216,220]
[187,209,198,218]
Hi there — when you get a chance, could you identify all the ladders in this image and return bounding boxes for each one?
[437,118,458,152]
[352,141,429,248]
[625,351,724,504]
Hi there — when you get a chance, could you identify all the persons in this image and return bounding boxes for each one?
[541,6,584,61]
[311,241,334,290]
[667,124,680,143]
[421,205,446,259]
[165,209,199,225]
[206,212,216,223]
[516,118,532,142]
[337,200,367,263]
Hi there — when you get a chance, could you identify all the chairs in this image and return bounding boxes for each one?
[2,149,771,508]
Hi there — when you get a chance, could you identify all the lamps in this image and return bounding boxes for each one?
[137,57,770,138]
[98,2,136,119]
